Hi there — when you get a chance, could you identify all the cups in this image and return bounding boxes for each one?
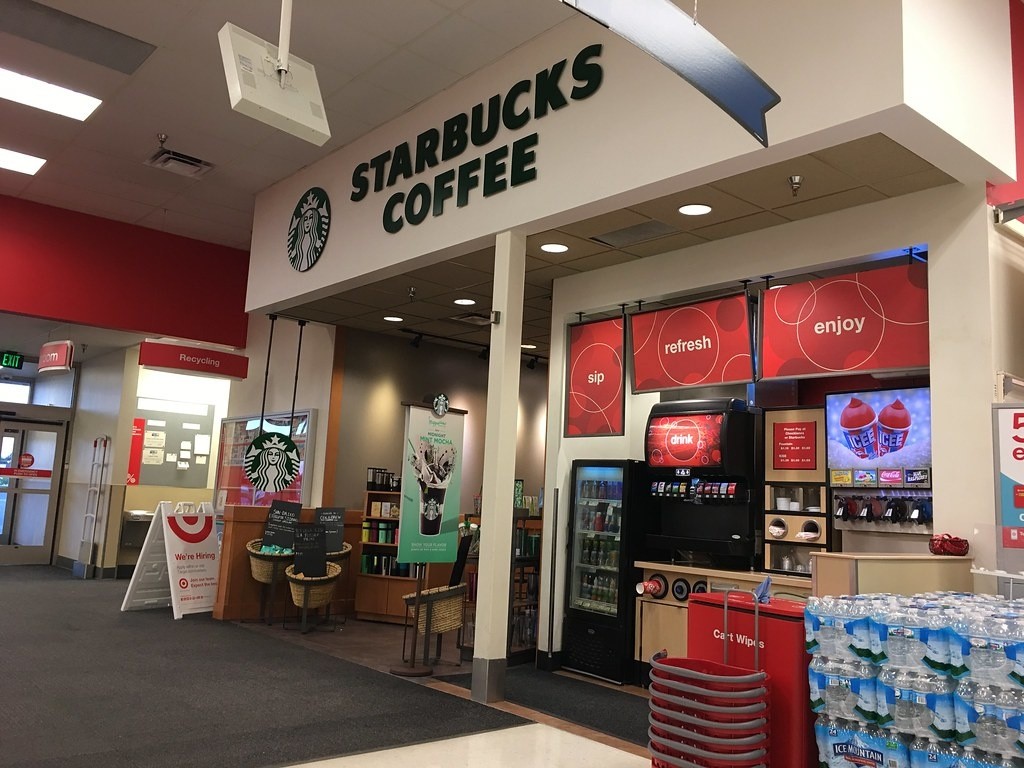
[790,502,799,511]
[635,580,661,595]
[840,414,878,461]
[528,534,540,554]
[878,419,912,458]
[420,483,448,536]
[776,497,790,510]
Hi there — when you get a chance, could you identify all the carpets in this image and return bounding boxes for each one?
[433,667,651,750]
[0,560,536,768]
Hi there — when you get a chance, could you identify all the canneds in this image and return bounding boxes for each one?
[588,511,605,531]
[581,538,618,605]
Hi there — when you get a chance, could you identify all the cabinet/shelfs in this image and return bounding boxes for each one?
[764,483,842,578]
[354,491,542,627]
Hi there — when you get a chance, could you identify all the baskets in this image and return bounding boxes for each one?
[285,561,342,609]
[245,538,352,585]
[401,582,468,636]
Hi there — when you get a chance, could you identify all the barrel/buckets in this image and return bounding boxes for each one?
[375,472,395,491]
[390,476,401,492]
[367,467,387,491]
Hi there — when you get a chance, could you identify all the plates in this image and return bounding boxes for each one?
[806,507,821,512]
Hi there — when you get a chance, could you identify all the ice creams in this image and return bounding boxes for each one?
[410,440,454,536]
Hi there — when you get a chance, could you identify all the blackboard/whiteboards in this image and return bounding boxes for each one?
[316,507,344,553]
[293,522,328,577]
[263,499,303,548]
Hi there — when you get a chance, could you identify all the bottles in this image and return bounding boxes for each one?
[523,609,537,647]
[473,495,481,517]
[387,523,394,543]
[804,591,1024,768]
[469,570,478,602]
[468,524,478,552]
[378,523,387,543]
[581,539,619,569]
[581,574,617,604]
[516,527,528,557]
[362,521,370,543]
[583,509,621,533]
[370,523,378,542]
[394,529,399,544]
[527,573,538,602]
[580,480,622,500]
[458,522,469,537]
